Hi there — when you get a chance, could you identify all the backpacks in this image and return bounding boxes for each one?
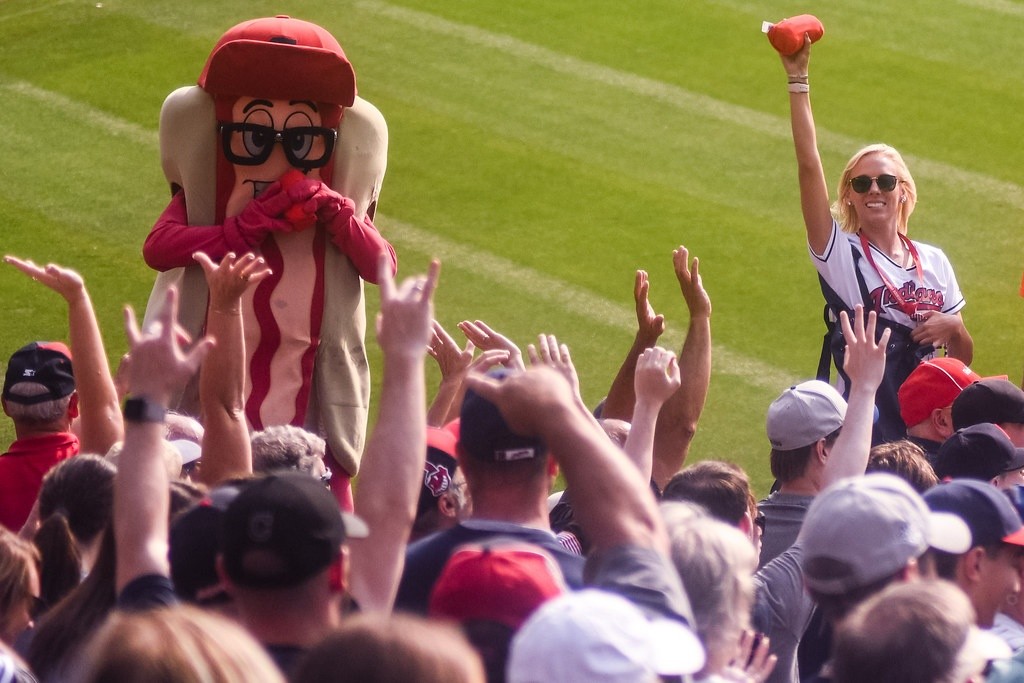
[811,244,935,440]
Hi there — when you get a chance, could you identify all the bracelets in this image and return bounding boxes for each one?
[788,74,809,93]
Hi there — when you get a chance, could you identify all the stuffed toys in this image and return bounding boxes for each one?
[142,15,396,591]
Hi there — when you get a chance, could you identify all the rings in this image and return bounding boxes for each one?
[32,277,38,281]
[432,346,437,351]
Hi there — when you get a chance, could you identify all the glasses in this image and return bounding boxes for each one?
[849,175,902,192]
[318,468,333,486]
[751,510,767,534]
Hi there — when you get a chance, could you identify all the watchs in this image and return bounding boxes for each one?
[122,397,164,422]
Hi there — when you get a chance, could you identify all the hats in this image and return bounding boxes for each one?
[105,438,202,479]
[922,478,1024,546]
[952,378,1024,432]
[429,539,564,631]
[416,417,461,520]
[938,422,1024,480]
[168,487,242,604]
[898,358,1008,427]
[461,368,547,461]
[802,473,973,593]
[3,341,74,403]
[504,588,705,682]
[767,380,879,450]
[221,472,369,586]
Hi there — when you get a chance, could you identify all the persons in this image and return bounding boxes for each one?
[0,245,1024,683]
[772,18,975,444]
